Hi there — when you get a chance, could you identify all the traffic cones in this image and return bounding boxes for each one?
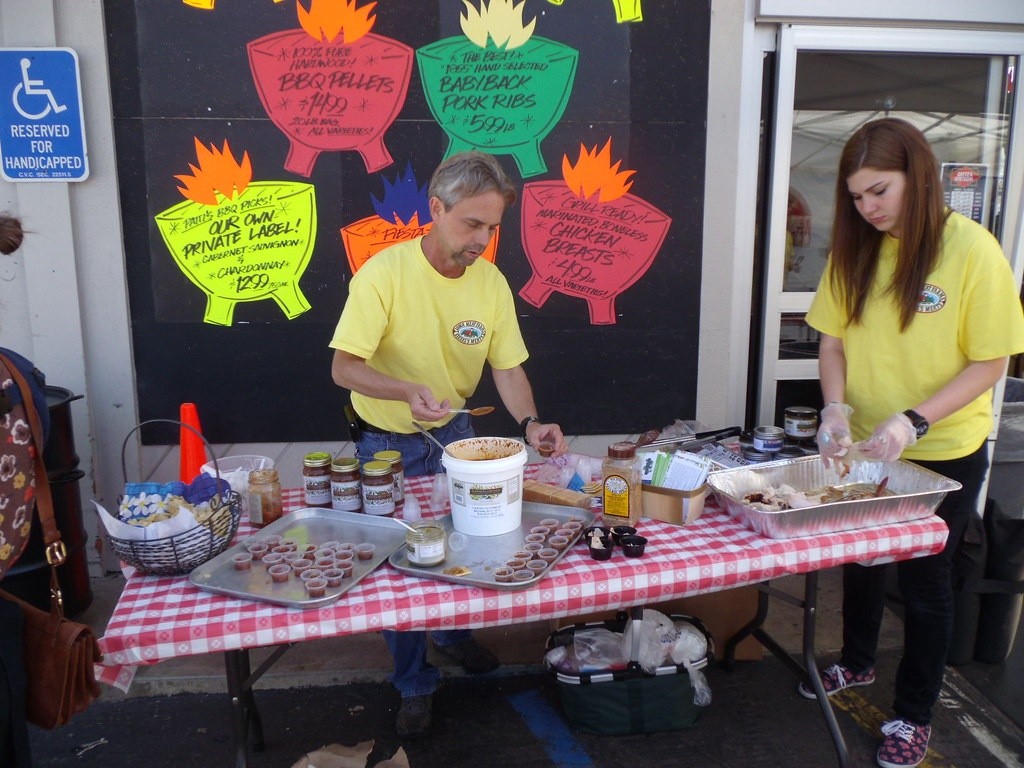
[180,402,209,485]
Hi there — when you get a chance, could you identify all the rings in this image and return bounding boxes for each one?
[879,436,887,443]
[822,433,829,444]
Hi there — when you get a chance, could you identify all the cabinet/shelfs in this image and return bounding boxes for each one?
[3,384,94,621]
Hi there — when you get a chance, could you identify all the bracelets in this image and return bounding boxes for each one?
[521,416,539,444]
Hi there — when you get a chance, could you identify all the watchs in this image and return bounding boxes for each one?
[902,409,929,440]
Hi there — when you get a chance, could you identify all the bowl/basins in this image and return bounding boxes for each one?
[538,445,554,457]
[231,532,376,597]
[610,525,637,546]
[199,455,275,514]
[492,516,586,582]
[589,540,614,560]
[584,527,610,545]
[620,535,647,558]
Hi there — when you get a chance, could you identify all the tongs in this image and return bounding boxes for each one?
[640,425,741,452]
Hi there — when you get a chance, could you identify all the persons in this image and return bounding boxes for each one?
[328,152,568,740]
[800,118,1024,768]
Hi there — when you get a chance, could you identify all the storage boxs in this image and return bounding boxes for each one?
[642,479,709,525]
[523,480,592,509]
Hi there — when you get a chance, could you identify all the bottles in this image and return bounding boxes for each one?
[406,520,446,567]
[737,405,820,464]
[247,468,282,527]
[601,440,642,527]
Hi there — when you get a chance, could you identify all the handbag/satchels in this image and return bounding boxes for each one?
[0,359,38,578]
[0,587,102,729]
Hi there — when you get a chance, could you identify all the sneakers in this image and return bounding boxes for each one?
[434,635,501,674]
[395,691,432,739]
[876,717,930,768]
[798,662,876,700]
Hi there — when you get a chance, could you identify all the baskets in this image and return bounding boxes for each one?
[103,419,242,578]
[545,604,714,737]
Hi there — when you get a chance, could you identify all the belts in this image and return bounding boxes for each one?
[362,423,391,434]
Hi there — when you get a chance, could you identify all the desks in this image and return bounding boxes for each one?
[95,443,949,767]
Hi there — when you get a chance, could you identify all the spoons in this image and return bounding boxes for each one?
[440,406,495,416]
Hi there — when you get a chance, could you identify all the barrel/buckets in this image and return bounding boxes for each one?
[0,384,94,620]
[442,437,527,536]
